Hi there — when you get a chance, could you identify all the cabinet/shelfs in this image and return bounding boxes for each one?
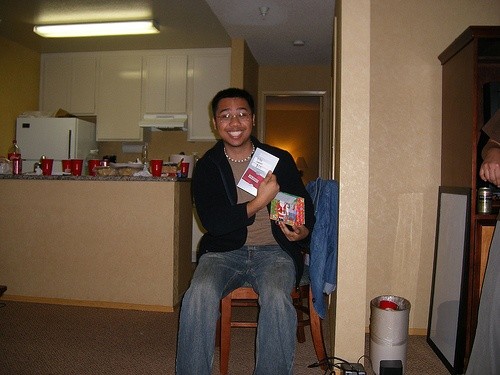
[38,46,232,141]
[437,24,500,357]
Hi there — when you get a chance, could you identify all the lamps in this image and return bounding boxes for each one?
[295,157,308,177]
[32,19,160,38]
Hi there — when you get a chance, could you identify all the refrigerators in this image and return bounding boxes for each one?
[16,116,98,175]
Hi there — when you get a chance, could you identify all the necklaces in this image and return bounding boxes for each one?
[223,140,255,162]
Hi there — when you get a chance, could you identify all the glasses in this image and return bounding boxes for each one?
[216,112,253,122]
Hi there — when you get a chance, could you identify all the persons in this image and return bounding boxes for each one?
[464,109,500,374]
[175,87,316,375]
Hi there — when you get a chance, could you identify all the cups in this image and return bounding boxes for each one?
[151,160,163,178]
[71,159,83,176]
[40,159,54,176]
[88,158,103,176]
[61,159,72,173]
[181,162,190,176]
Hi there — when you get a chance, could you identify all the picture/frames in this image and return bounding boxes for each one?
[426,186,474,375]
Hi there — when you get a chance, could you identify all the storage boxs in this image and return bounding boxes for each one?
[270,190,306,226]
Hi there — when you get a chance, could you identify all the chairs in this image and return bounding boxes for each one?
[215,177,339,375]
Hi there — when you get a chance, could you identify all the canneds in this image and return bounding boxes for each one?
[478,186,492,214]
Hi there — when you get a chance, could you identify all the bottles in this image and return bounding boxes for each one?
[143,144,147,158]
[85,149,99,175]
[8,140,21,161]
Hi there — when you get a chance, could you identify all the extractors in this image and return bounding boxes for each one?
[138,113,188,128]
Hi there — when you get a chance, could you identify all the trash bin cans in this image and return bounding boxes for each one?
[369,295,412,375]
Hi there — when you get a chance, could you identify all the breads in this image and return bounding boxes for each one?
[98,168,132,176]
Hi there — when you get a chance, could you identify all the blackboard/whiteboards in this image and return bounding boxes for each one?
[426,184,472,375]
